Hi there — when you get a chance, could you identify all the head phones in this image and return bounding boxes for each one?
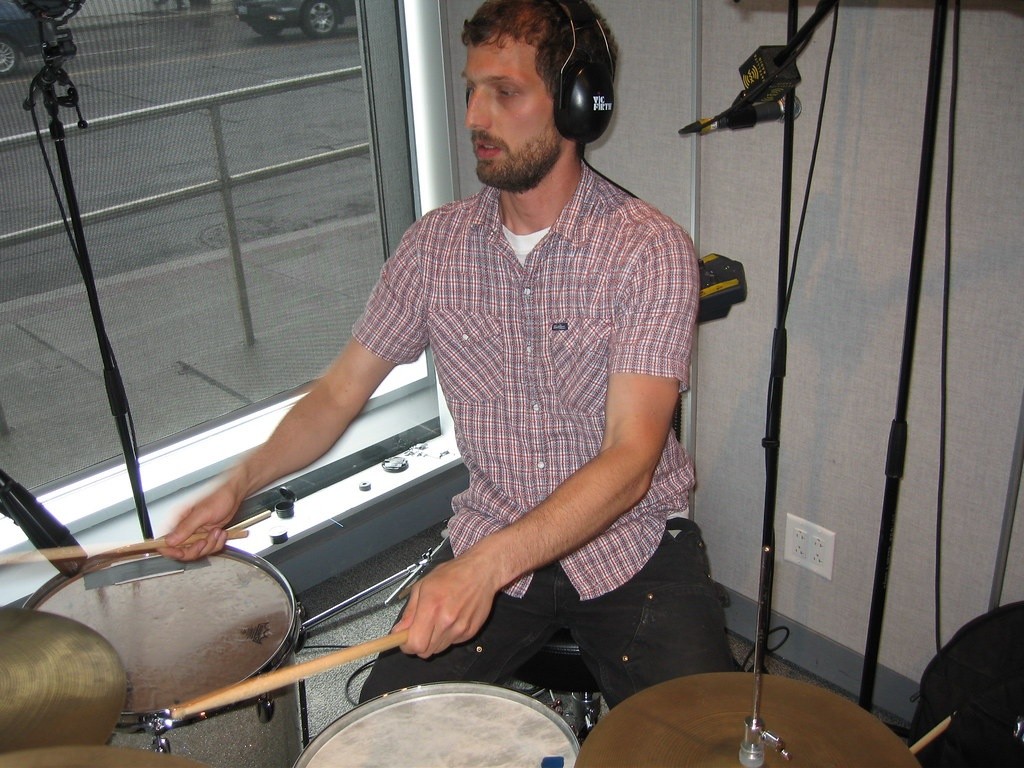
[554,0,614,144]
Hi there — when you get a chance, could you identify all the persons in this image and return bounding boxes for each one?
[158,0,737,710]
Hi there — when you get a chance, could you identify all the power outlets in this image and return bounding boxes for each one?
[784,513,836,580]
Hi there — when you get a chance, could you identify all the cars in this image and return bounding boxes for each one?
[232,0,355,41]
[0,0,57,79]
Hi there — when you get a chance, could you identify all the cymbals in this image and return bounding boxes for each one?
[574,669,918,767]
[0,606,211,768]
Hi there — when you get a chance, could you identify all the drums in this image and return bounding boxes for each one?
[294,675,583,768]
[18,540,306,768]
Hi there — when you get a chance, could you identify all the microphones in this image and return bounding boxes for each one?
[12,0,85,64]
[682,96,802,135]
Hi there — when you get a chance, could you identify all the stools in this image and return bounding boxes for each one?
[516,631,601,747]
